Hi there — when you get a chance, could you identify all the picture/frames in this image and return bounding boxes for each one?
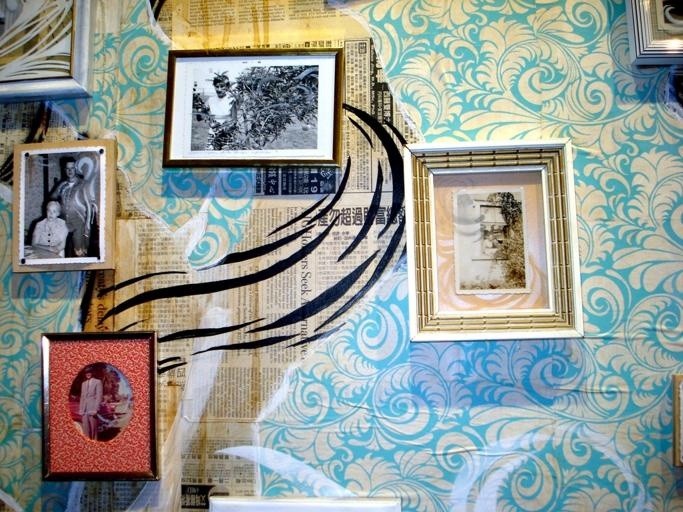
[39,329,161,483]
[0,0,92,104]
[161,46,346,169]
[403,138,584,342]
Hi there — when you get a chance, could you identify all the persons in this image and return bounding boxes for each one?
[196,75,239,151]
[77,367,104,442]
[47,156,92,258]
[28,200,69,260]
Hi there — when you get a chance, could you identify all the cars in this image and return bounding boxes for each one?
[64,396,130,442]
